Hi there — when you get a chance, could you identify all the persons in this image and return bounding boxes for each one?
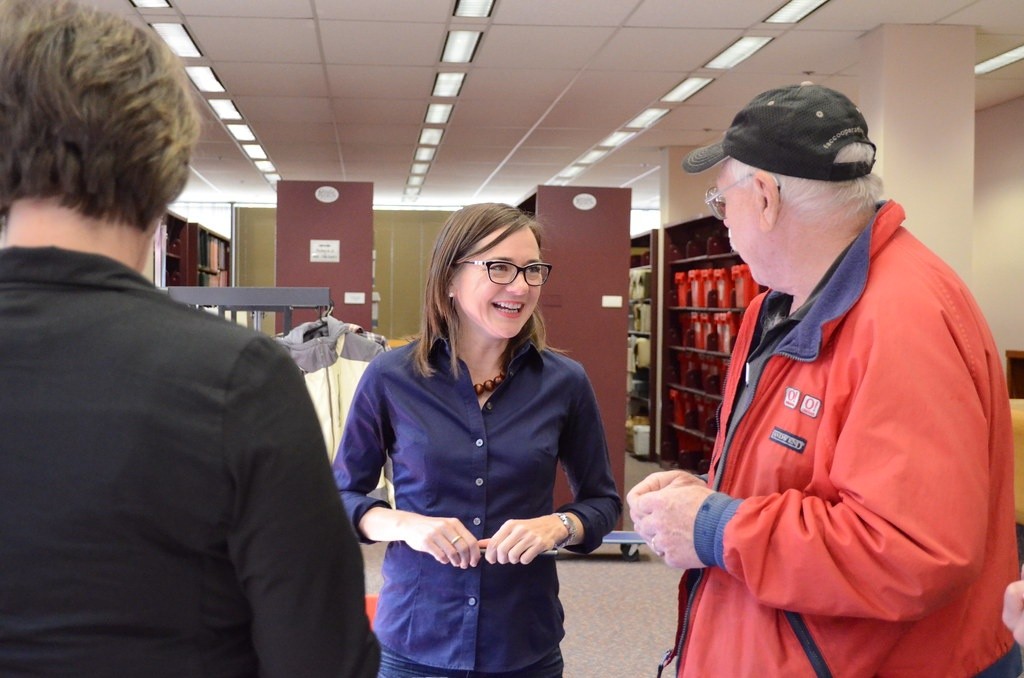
[625,86,1024,678]
[0,0,382,678]
[330,203,625,677]
[999,565,1024,652]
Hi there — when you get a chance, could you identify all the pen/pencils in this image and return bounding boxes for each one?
[480,548,560,557]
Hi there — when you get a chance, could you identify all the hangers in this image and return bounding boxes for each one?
[303,299,335,340]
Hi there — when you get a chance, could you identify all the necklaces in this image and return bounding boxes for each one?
[473,367,508,395]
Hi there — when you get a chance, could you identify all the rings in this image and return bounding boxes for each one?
[651,537,664,556]
[451,535,462,544]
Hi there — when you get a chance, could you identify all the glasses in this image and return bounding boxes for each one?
[464,260,552,286]
[705,170,780,220]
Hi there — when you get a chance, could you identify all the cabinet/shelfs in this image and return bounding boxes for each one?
[140,208,188,287]
[654,215,770,476]
[624,228,658,463]
[518,185,632,532]
[188,222,231,324]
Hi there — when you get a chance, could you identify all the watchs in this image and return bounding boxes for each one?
[553,512,577,549]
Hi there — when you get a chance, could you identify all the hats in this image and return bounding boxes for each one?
[681,81,877,181]
